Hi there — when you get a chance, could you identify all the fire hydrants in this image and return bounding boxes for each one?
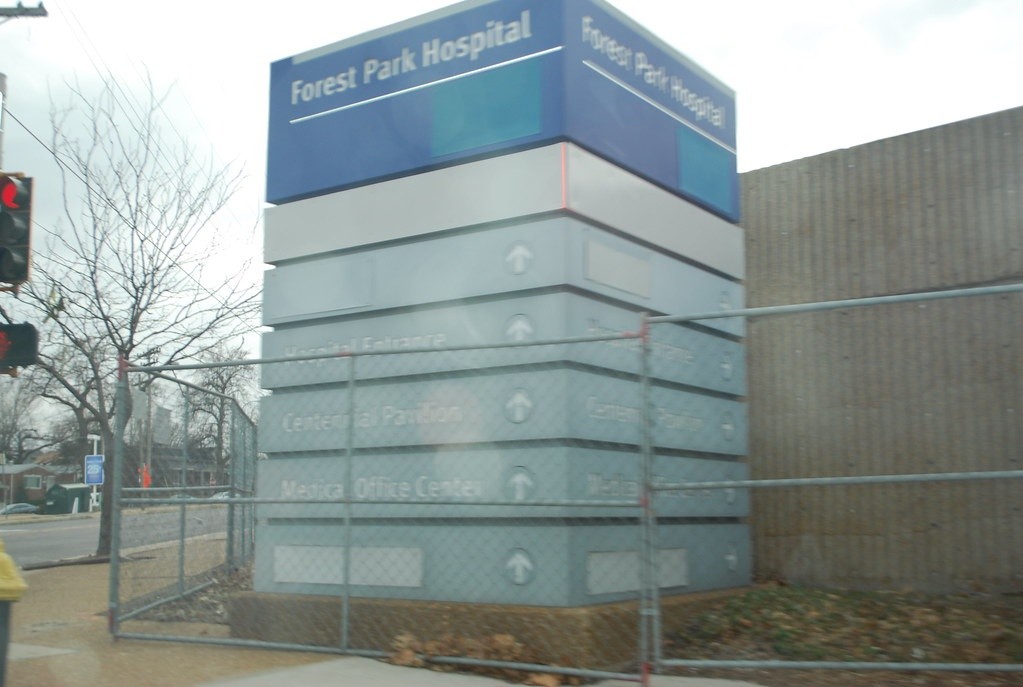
[0,539,29,687]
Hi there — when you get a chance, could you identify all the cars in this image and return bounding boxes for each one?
[170,493,198,499]
[0,502,41,514]
[211,491,240,499]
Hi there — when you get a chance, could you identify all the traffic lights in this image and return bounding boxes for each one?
[0,174,35,286]
[0,321,39,370]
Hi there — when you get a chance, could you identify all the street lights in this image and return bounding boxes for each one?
[84,433,102,508]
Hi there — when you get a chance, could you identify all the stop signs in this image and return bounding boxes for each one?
[210,479,216,484]
